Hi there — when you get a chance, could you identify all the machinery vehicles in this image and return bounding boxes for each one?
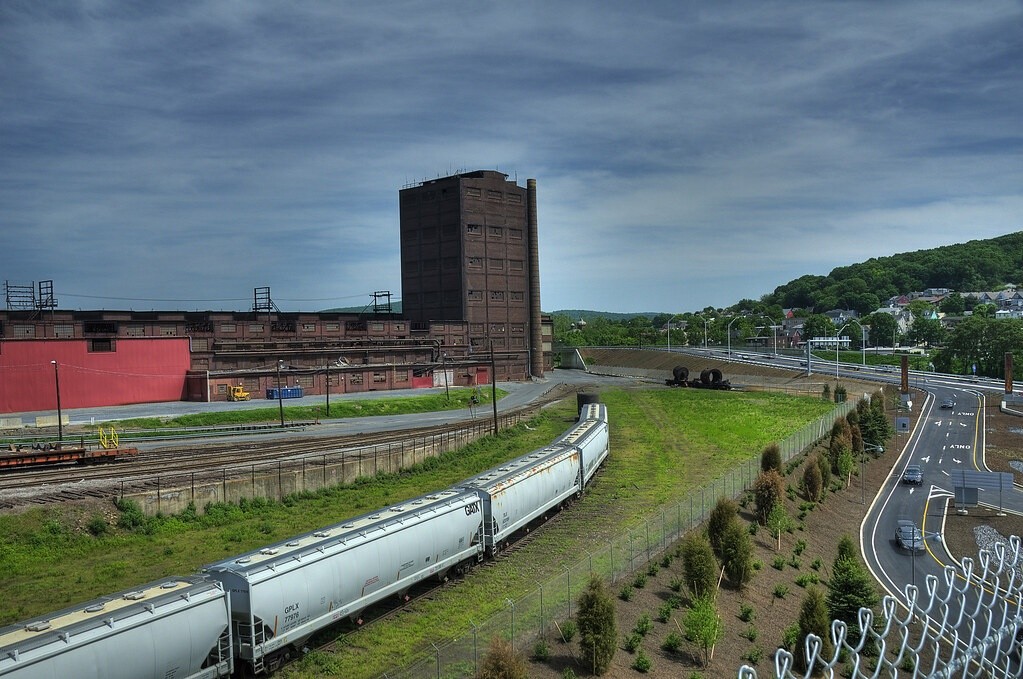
[227,385,251,402]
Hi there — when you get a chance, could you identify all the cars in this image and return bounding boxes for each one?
[844,366,860,371]
[966,375,978,383]
[722,350,732,355]
[800,362,815,369]
[742,354,756,360]
[895,520,926,556]
[763,354,774,359]
[875,365,901,373]
[939,400,953,409]
[903,465,923,486]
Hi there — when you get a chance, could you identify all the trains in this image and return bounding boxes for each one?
[0,403,610,679]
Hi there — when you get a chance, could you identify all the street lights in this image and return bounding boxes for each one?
[668,314,677,353]
[852,320,865,365]
[51,353,450,440]
[862,441,883,505]
[696,314,707,349]
[765,316,776,355]
[728,317,739,360]
[837,324,850,379]
